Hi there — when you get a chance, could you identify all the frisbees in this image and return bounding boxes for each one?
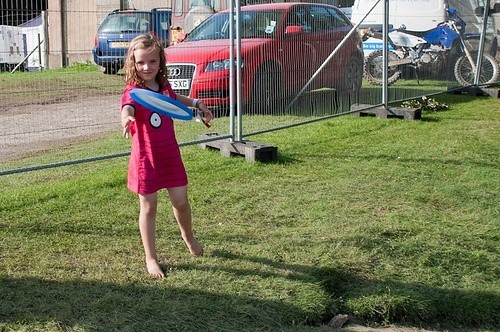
[128,88,193,121]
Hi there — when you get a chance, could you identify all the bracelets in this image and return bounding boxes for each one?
[196,100,203,108]
[192,99,198,107]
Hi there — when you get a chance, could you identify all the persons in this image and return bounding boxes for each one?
[120,33,214,279]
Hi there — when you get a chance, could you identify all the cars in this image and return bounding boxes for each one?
[163,2,364,115]
[94,7,172,74]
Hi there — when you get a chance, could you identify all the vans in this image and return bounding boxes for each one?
[351,0,500,58]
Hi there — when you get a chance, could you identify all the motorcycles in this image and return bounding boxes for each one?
[364,6,499,87]
